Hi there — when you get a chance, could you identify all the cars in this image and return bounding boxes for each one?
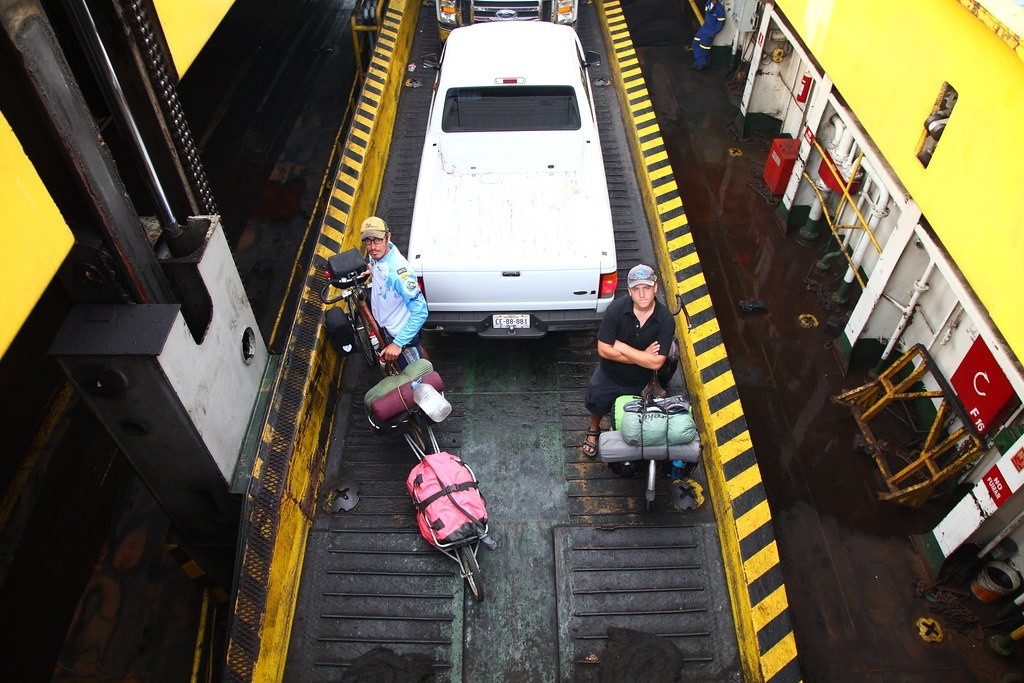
[431,0,592,44]
[403,19,617,339]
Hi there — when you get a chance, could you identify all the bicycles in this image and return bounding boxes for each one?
[319,248,490,607]
[625,294,686,513]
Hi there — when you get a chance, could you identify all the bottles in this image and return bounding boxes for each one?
[411,381,452,423]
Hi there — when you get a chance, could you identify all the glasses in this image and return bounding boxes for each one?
[361,234,388,246]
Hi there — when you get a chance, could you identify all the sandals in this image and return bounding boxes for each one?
[583,423,601,456]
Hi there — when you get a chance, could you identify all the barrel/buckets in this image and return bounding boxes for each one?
[971,560,1020,604]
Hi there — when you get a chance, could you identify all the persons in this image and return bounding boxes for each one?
[690,0,725,70]
[582,264,675,456]
[360,216,429,369]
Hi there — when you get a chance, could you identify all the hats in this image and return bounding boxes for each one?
[360,216,389,243]
[627,264,656,289]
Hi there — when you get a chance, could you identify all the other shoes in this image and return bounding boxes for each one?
[692,63,708,70]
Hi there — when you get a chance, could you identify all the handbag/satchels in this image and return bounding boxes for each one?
[407,451,488,545]
[598,393,705,480]
[363,360,444,434]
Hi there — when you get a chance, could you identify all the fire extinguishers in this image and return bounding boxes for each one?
[796,70,813,103]
